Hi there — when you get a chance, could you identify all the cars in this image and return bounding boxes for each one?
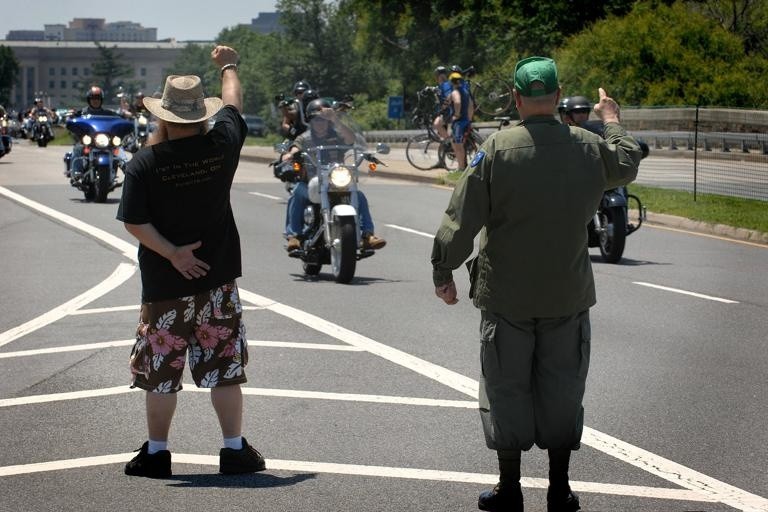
[240,113,265,138]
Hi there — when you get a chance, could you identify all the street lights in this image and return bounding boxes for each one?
[33,90,49,100]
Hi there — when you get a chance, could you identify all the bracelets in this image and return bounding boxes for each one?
[221,63,237,74]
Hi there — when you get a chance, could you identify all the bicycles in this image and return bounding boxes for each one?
[405,65,514,172]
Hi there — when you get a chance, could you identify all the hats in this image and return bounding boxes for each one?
[140,72,226,124]
[512,55,562,98]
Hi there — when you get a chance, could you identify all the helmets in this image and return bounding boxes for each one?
[433,64,466,82]
[558,96,592,114]
[293,80,333,118]
[86,86,105,109]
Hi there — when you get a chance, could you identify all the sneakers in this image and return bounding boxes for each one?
[219,437,266,474]
[124,440,175,478]
[359,233,386,249]
[545,470,580,512]
[287,236,301,252]
[478,477,525,512]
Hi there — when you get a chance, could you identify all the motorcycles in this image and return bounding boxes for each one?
[268,94,390,283]
[571,120,650,265]
[0,105,155,203]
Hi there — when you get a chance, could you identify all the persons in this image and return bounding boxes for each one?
[558,98,569,125]
[430,55,643,512]
[270,79,390,285]
[117,46,266,480]
[564,96,604,140]
[0,85,164,192]
[426,65,476,171]
